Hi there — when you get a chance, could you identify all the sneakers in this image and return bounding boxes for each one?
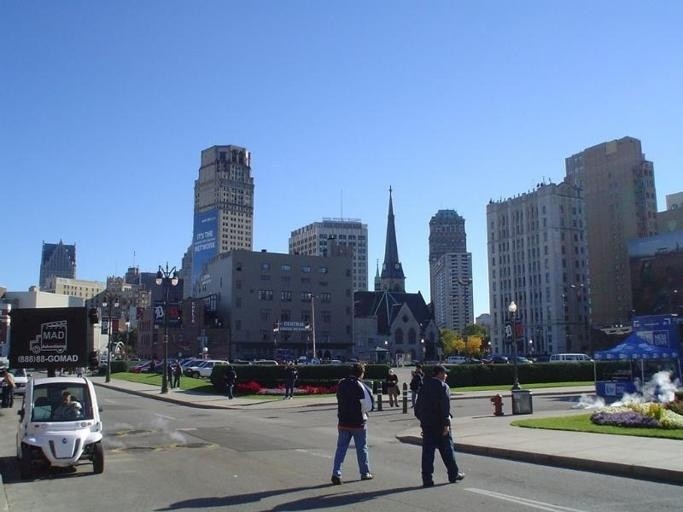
[330,475,343,486]
[360,470,374,481]
[448,469,465,484]
[422,475,434,488]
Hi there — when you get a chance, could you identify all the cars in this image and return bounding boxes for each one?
[638,245,675,267]
[446,353,538,364]
[16,375,104,481]
[0,365,33,396]
[129,355,364,378]
[92,352,115,376]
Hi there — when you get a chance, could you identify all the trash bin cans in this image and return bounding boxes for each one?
[511,390,532,415]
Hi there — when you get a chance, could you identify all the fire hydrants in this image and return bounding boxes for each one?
[491,394,505,416]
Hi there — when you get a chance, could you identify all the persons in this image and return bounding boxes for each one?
[1,367,17,408]
[224,366,238,400]
[76,366,85,377]
[414,364,465,488]
[331,363,375,485]
[54,391,82,419]
[283,363,298,401]
[410,365,425,408]
[151,359,156,372]
[167,359,183,389]
[386,368,400,407]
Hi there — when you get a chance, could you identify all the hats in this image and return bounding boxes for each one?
[434,364,451,375]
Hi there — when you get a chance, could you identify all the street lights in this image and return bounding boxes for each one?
[570,282,584,352]
[310,294,318,358]
[487,341,492,357]
[154,261,178,393]
[101,290,119,383]
[508,300,521,391]
[528,339,533,356]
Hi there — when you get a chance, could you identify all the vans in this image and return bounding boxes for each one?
[549,353,593,362]
